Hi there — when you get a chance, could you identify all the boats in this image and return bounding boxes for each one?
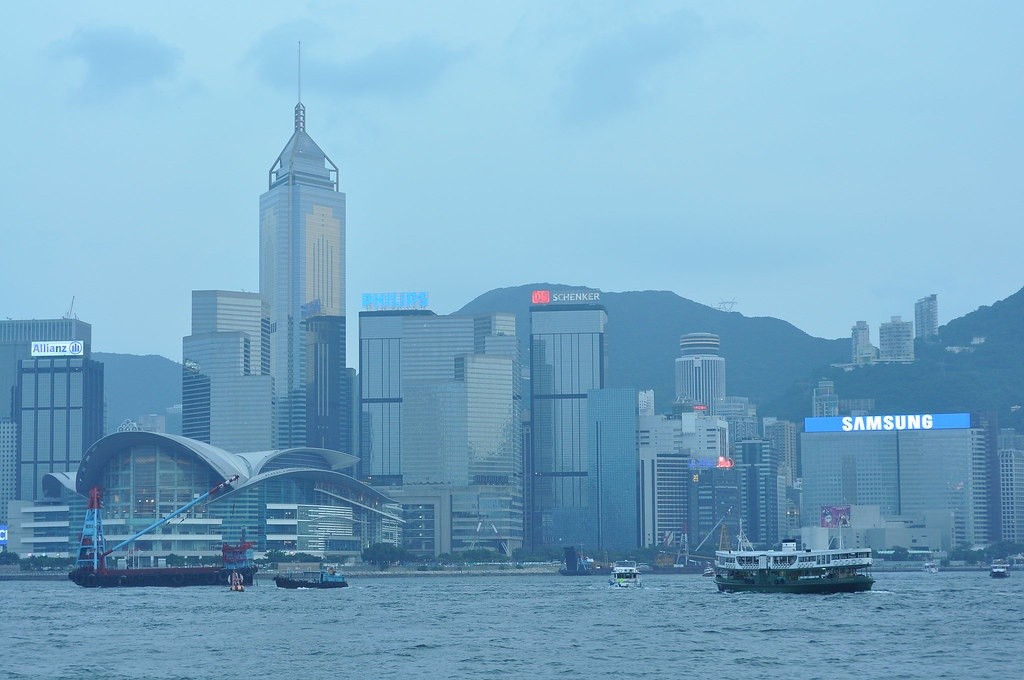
[924,563,938,573]
[558,546,613,576]
[69,474,259,588]
[229,585,245,591]
[272,566,348,588]
[715,516,875,594]
[609,560,641,587]
[991,559,1010,577]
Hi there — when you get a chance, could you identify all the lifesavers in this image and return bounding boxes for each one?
[718,584,734,592]
[775,578,783,584]
[744,578,754,584]
